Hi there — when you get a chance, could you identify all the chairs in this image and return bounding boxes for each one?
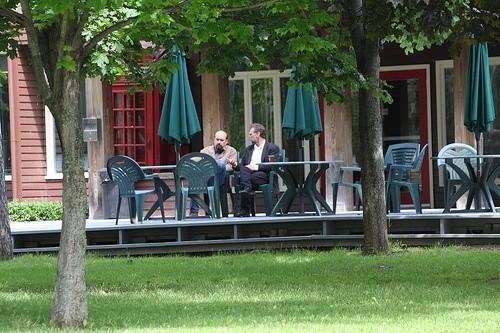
[331,143,428,215]
[437,143,496,213]
[173,149,285,221]
[106,155,165,224]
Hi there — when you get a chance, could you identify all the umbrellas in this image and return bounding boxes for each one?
[464,32,496,210]
[157,46,201,217]
[282,65,323,213]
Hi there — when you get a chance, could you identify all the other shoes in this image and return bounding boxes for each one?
[239,188,254,197]
[237,210,249,217]
[189,212,197,216]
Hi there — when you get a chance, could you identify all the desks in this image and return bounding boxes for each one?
[140,165,213,220]
[256,160,345,217]
[430,155,500,213]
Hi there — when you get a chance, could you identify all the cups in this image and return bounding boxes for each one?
[268,155,275,162]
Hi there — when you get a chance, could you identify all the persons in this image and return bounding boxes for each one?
[228,123,279,218]
[189,131,238,216]
